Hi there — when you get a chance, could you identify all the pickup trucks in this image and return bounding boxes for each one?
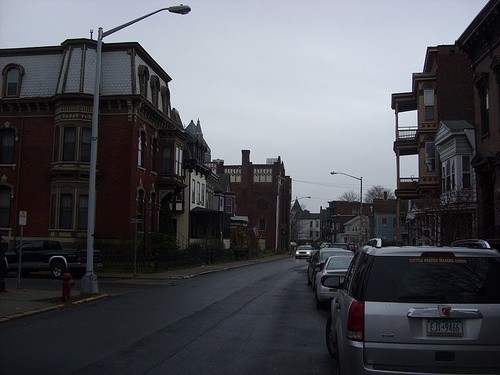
[5,240,104,281]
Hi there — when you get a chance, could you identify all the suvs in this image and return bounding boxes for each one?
[319,242,350,251]
[325,239,500,375]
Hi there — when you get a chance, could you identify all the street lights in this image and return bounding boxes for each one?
[78,3,192,296]
[288,196,312,255]
[329,171,364,245]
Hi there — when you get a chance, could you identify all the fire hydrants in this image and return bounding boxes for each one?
[59,272,75,302]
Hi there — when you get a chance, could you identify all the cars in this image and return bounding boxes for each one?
[306,248,355,291]
[315,256,361,307]
[295,245,315,259]
